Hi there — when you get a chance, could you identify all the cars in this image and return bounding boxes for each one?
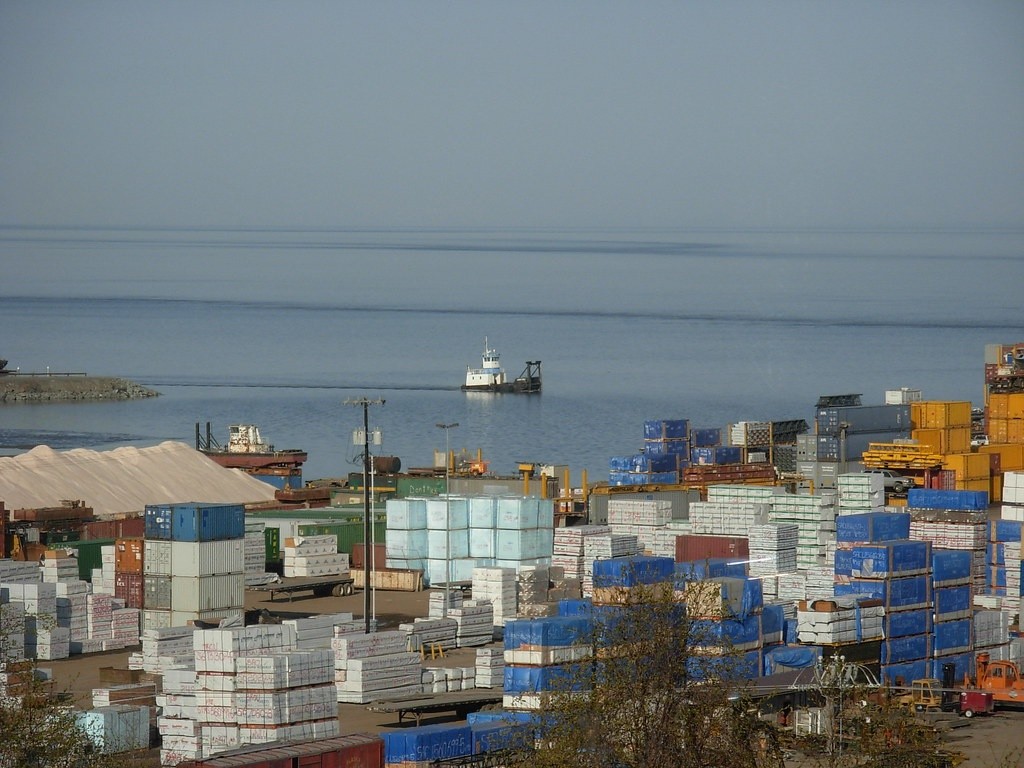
[970,434,989,446]
[861,468,915,493]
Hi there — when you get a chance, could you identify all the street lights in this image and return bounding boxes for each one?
[432,420,460,609]
[832,655,845,756]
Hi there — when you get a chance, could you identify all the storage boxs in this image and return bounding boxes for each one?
[0,392,1024,768]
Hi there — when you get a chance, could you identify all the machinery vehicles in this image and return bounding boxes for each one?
[964,651,1024,710]
[897,679,942,718]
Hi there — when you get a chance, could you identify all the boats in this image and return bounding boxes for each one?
[460,333,543,393]
[192,419,310,470]
